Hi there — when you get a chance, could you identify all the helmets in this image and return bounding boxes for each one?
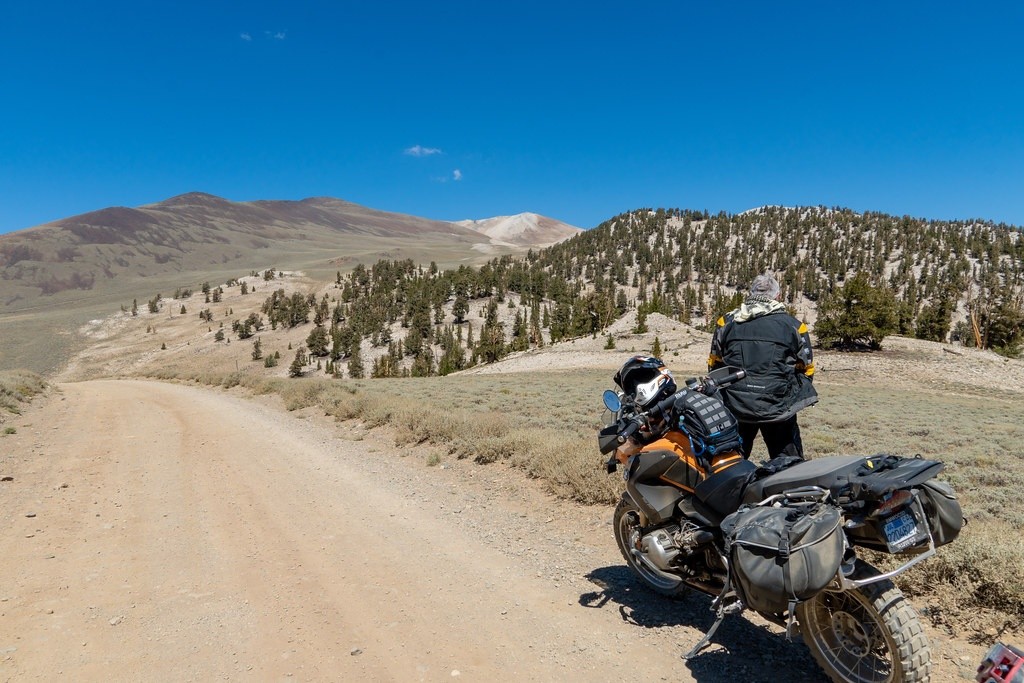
[614,355,677,409]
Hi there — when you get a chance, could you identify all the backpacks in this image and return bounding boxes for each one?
[667,384,743,473]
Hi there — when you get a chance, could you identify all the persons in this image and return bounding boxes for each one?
[707,275,819,462]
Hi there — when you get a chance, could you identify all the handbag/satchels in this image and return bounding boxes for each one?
[720,502,845,599]
[867,453,963,547]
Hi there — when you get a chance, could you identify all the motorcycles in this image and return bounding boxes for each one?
[597,356,968,683]
